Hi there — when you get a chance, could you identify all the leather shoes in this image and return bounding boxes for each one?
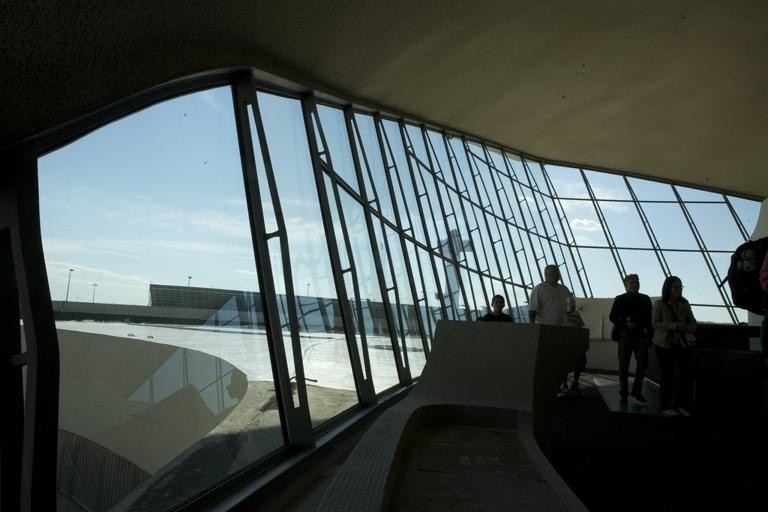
[618,391,648,403]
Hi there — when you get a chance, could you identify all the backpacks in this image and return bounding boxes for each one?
[727,236,768,315]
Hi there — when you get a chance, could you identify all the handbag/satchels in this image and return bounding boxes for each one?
[612,324,620,343]
[680,333,697,348]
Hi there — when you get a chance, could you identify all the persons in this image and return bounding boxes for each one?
[484,295,512,321]
[608,274,696,409]
[528,265,585,388]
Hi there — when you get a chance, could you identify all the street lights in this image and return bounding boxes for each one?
[93,283,98,303]
[307,284,310,296]
[66,268,74,301]
[188,276,192,287]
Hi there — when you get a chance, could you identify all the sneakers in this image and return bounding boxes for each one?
[661,407,691,417]
